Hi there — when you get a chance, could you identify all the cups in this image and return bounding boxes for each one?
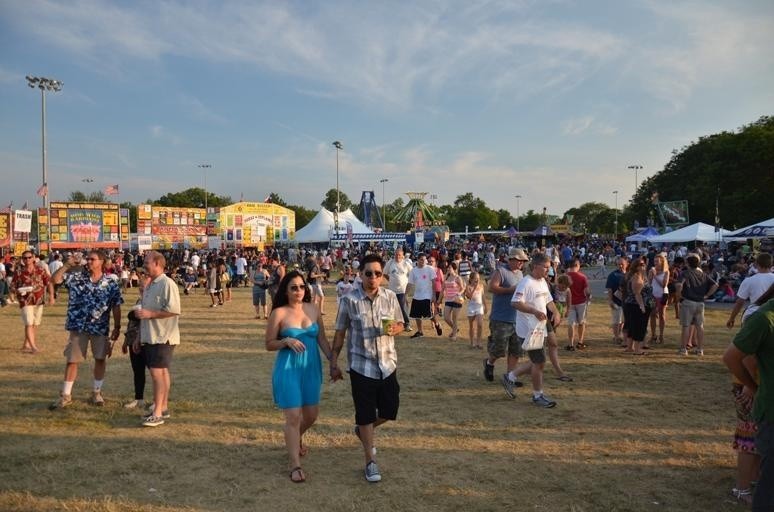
[382,317,395,335]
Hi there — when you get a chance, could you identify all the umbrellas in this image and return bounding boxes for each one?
[647,222,731,242]
[722,218,773,238]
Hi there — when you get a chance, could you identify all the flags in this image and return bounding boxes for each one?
[0,204,10,213]
[20,202,27,210]
[105,185,118,195]
[36,184,47,197]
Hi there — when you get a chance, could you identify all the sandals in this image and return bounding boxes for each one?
[731,486,754,502]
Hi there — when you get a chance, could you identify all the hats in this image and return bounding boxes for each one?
[503,247,530,261]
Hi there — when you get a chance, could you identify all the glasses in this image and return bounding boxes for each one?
[365,271,382,276]
[23,256,32,259]
[290,285,305,290]
[85,257,100,261]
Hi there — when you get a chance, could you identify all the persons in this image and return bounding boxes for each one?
[403,252,443,338]
[382,247,413,333]
[158,242,395,319]
[722,298,774,512]
[134,249,182,426]
[397,236,637,279]
[622,256,635,342]
[264,269,344,484]
[441,262,466,338]
[552,275,571,323]
[329,254,404,486]
[463,270,489,350]
[0,234,143,408]
[544,266,574,383]
[605,258,629,346]
[122,271,153,407]
[501,251,562,406]
[568,260,592,353]
[427,256,447,316]
[483,247,529,387]
[647,241,774,356]
[624,256,653,355]
[729,282,774,503]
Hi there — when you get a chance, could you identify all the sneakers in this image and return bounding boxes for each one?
[141,415,165,427]
[434,322,442,336]
[364,460,382,483]
[122,399,146,409]
[696,346,704,357]
[482,358,494,382]
[48,390,73,410]
[531,393,556,408]
[576,342,587,349]
[140,408,172,420]
[515,381,523,388]
[564,345,575,351]
[90,391,106,406]
[410,331,424,339]
[404,325,413,331]
[499,373,516,400]
[148,402,157,412]
[677,345,688,356]
[354,425,378,457]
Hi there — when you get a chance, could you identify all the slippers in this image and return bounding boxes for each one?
[290,465,307,484]
[557,376,574,383]
[632,351,648,356]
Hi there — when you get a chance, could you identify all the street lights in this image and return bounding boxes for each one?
[27,76,63,306]
[516,194,521,234]
[84,178,122,255]
[380,178,386,234]
[198,163,211,255]
[613,189,619,236]
[629,164,646,227]
[333,141,340,234]
